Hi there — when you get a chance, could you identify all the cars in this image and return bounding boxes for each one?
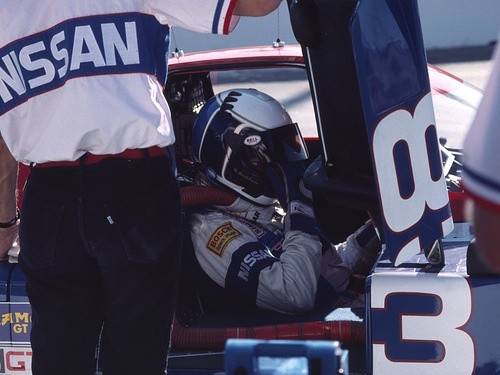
[1,43,499,375]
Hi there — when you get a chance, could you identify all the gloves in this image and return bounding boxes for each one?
[354,219,382,257]
[278,162,321,236]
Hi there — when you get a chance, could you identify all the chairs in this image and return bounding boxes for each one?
[171,176,366,350]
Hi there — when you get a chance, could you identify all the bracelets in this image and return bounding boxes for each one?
[0,218,17,228]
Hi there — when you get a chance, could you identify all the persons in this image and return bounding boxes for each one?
[0,0,283,374]
[190,88,379,316]
[458,44,500,274]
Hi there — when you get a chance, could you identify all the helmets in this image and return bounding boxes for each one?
[189,88,309,206]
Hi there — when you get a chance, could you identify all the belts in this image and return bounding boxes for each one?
[34,146,169,168]
